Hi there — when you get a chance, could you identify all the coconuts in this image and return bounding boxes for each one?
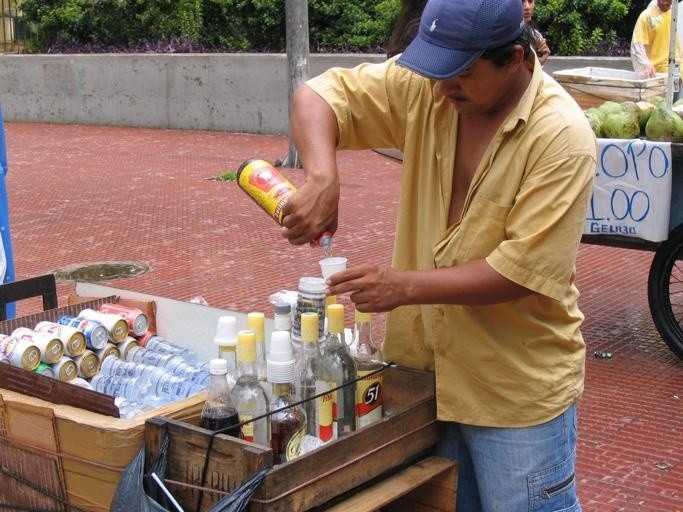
[584,95,683,142]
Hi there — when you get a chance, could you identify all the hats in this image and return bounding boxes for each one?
[396,0,525,79]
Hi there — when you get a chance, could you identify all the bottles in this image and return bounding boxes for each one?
[234,157,333,249]
[204,276,385,462]
[69,334,214,424]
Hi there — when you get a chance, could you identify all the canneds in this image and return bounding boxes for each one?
[0,302,158,383]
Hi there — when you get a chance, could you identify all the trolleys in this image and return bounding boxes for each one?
[572,138,682,359]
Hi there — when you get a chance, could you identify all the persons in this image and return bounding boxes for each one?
[281,1,598,512]
[521,0,552,71]
[630,2,683,106]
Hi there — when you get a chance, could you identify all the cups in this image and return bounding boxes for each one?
[320,257,347,283]
